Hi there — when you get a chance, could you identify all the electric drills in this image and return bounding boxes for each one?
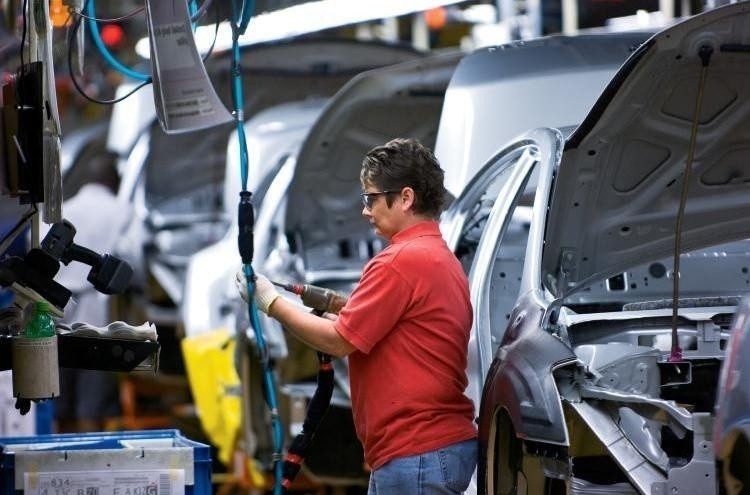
[269,280,349,365]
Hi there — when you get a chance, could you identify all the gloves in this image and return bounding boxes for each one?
[235,272,281,316]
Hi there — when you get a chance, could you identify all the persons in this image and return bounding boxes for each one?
[236,137,479,494]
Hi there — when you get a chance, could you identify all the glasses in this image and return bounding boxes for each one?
[361,188,402,212]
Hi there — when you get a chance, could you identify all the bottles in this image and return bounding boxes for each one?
[25,301,56,341]
